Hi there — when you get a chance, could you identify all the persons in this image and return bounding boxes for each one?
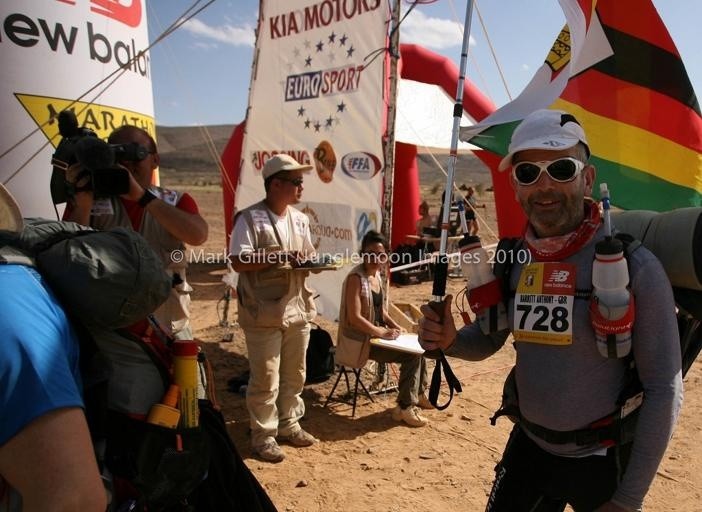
[62,125,209,340]
[0,240,111,511]
[227,154,323,460]
[340,228,438,427]
[418,201,433,229]
[464,186,485,236]
[416,110,683,512]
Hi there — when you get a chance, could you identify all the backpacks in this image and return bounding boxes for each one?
[0,217,212,509]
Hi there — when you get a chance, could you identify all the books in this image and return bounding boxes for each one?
[369,331,429,358]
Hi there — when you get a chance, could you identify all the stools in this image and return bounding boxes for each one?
[326,344,377,418]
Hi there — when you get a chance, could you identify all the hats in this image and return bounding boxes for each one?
[262,154,313,179]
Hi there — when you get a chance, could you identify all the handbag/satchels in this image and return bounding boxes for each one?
[306,321,335,385]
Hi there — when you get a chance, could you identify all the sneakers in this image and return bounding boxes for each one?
[279,428,314,446]
[251,440,284,462]
[391,404,428,426]
[419,394,434,409]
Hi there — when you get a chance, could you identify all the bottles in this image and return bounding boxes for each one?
[148,383,183,430]
[173,338,201,426]
[456,238,514,335]
[590,238,635,358]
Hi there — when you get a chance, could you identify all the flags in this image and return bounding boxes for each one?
[455,2,702,240]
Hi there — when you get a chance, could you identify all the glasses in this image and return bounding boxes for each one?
[511,157,585,186]
[278,177,302,186]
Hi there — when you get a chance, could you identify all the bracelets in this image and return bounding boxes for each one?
[136,187,156,208]
